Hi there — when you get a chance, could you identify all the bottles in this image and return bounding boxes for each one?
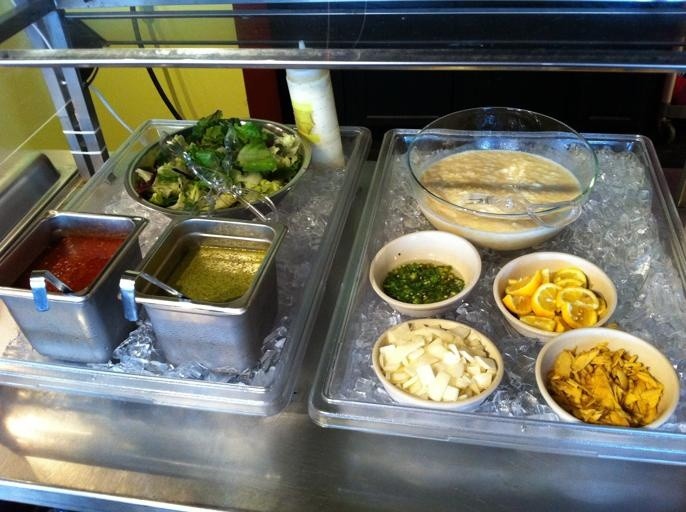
[282,40,348,173]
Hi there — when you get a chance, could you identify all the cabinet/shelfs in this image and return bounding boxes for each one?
[0,1,686,510]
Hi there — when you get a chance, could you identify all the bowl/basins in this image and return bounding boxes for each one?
[532,323,680,428]
[404,104,601,248]
[371,317,506,413]
[123,115,314,220]
[367,229,484,317]
[490,251,620,337]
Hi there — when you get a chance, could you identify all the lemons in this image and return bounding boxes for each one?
[503,266,607,333]
